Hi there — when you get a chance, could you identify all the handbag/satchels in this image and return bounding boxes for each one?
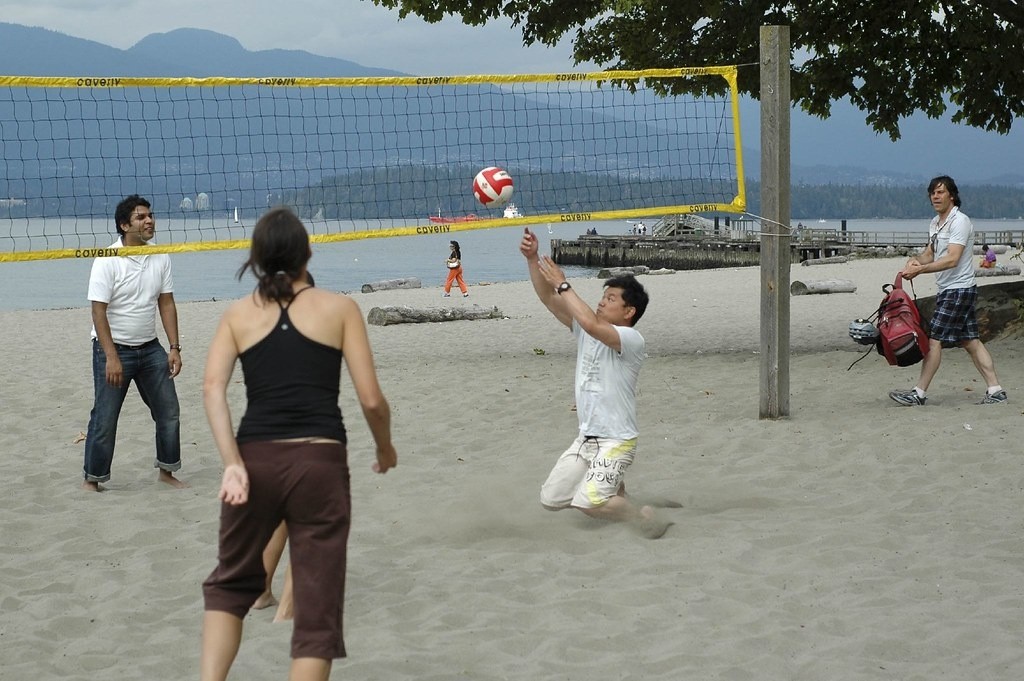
[447,250,459,269]
[984,260,989,268]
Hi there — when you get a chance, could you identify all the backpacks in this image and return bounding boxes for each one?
[876,271,932,367]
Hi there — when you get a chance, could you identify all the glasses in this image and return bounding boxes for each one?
[930,233,938,253]
[449,244,454,247]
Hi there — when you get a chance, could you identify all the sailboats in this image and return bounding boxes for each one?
[233,206,239,225]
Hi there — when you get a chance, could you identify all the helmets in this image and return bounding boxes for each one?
[849,318,880,345]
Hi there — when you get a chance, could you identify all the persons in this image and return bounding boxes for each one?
[628,220,646,236]
[442,240,469,298]
[198,204,397,681]
[889,174,1010,407]
[82,195,185,491]
[587,228,598,235]
[980,244,997,268]
[519,225,668,540]
[248,519,296,625]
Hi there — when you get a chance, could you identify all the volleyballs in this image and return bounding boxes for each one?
[473,165,513,208]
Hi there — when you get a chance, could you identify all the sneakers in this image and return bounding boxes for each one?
[889,387,928,406]
[973,389,1007,405]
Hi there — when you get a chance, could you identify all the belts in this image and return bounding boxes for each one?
[94,338,158,350]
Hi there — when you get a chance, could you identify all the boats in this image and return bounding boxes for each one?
[429,213,495,223]
[502,202,523,219]
[651,214,745,239]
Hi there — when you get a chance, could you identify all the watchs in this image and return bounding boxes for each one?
[557,281,571,295]
[170,344,182,353]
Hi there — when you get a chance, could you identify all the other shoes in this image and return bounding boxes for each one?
[443,292,450,297]
[464,293,469,297]
